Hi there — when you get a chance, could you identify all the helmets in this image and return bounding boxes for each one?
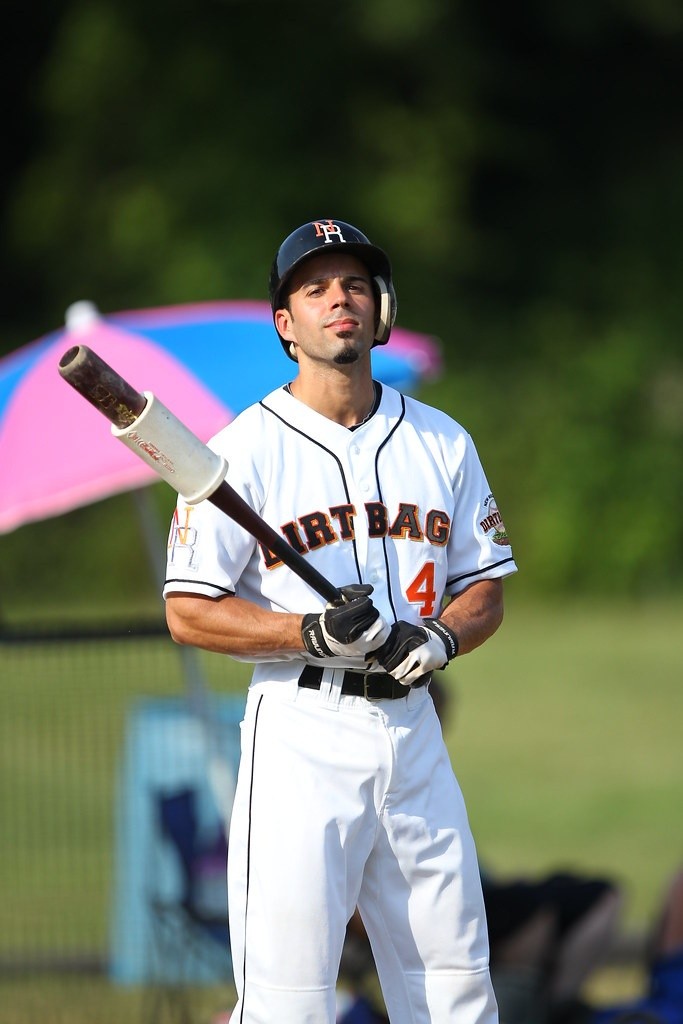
[271,219,398,362]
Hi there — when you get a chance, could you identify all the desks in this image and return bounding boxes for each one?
[110,694,260,986]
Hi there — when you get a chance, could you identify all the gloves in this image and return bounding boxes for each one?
[299,583,392,659]
[366,620,458,687]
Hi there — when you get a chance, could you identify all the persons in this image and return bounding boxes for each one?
[345,676,683,1024]
[164,220,517,1024]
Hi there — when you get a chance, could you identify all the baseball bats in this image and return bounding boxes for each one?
[58,344,350,607]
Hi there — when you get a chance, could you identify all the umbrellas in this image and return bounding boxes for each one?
[0,300,440,703]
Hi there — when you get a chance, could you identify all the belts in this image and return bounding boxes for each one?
[296,664,411,702]
